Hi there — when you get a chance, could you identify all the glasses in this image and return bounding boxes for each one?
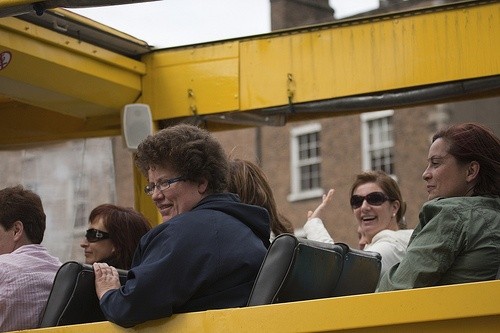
[85,228,111,243]
[350,191,395,208]
[144,174,193,197]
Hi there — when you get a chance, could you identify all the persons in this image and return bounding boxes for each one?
[0,184,63,333]
[93,125,272,328]
[81,203,152,271]
[222,159,294,243]
[303,170,415,293]
[379,123,500,292]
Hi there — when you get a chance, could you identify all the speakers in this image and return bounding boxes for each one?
[122,103,153,150]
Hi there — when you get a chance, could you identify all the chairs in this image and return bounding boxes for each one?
[246,232,384,308]
[38,260,128,328]
